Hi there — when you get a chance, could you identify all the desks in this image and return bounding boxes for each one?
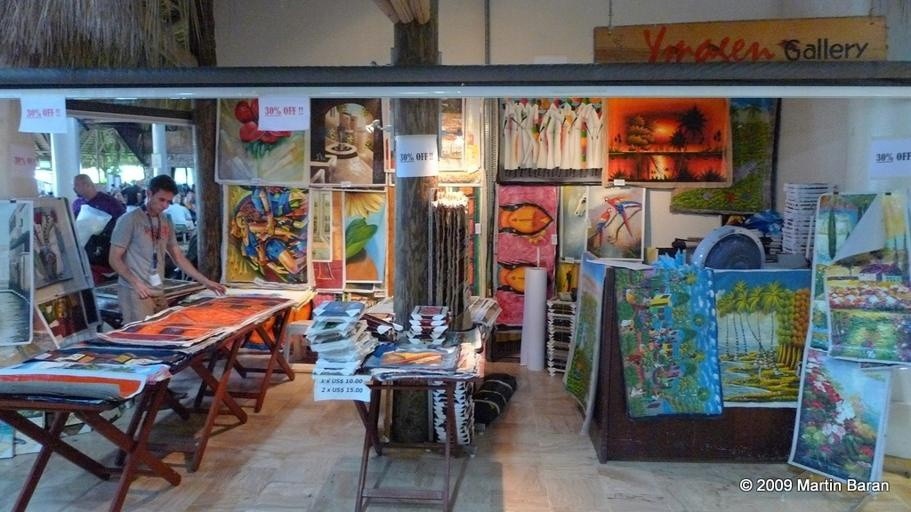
[2,289,490,509]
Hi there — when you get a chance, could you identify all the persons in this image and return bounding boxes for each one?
[109,175,229,410]
[38,176,198,232]
[68,174,128,288]
